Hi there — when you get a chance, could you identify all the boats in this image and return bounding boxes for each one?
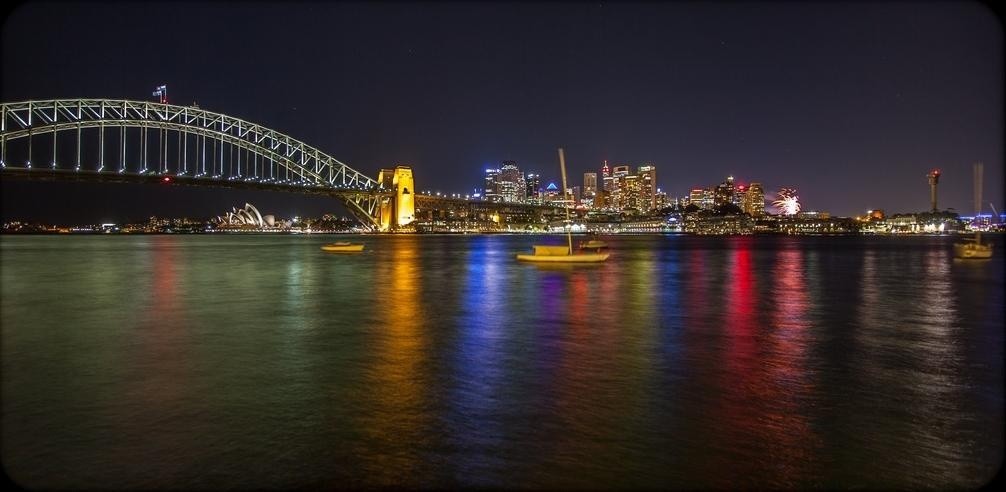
[319,241,365,252]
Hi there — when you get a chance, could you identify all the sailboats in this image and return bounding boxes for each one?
[516,147,611,263]
[950,160,994,257]
